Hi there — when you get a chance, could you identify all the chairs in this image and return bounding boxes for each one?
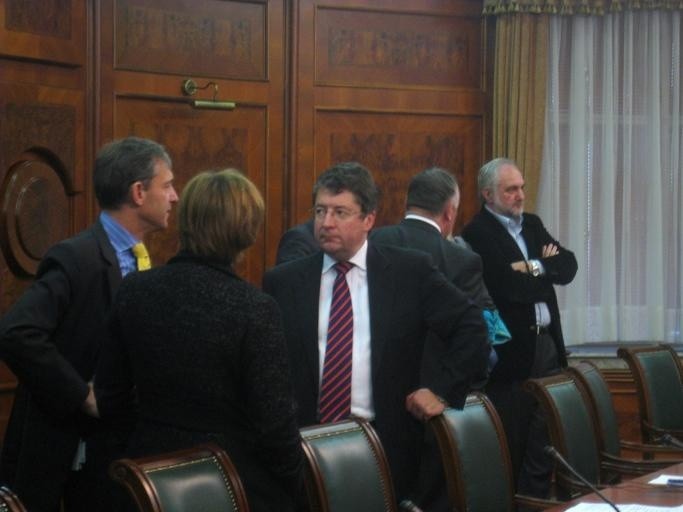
[299,418,395,511]
[430,392,560,511]
[617,343,683,461]
[562,358,679,486]
[110,443,250,512]
[0,486,25,512]
[523,375,605,501]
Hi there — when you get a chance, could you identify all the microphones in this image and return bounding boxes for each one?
[660,431,683,453]
[543,443,621,511]
[398,496,424,512]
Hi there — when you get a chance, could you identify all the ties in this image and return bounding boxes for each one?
[319,261,359,426]
[128,240,152,272]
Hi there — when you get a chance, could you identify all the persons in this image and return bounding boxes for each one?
[263,160,485,488]
[368,168,485,414]
[464,158,577,510]
[277,221,315,261]
[3,136,180,510]
[74,170,298,512]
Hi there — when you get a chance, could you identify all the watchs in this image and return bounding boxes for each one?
[531,262,540,278]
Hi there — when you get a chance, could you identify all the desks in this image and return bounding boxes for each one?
[539,461,683,512]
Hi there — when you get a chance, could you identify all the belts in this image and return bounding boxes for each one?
[533,324,555,335]
